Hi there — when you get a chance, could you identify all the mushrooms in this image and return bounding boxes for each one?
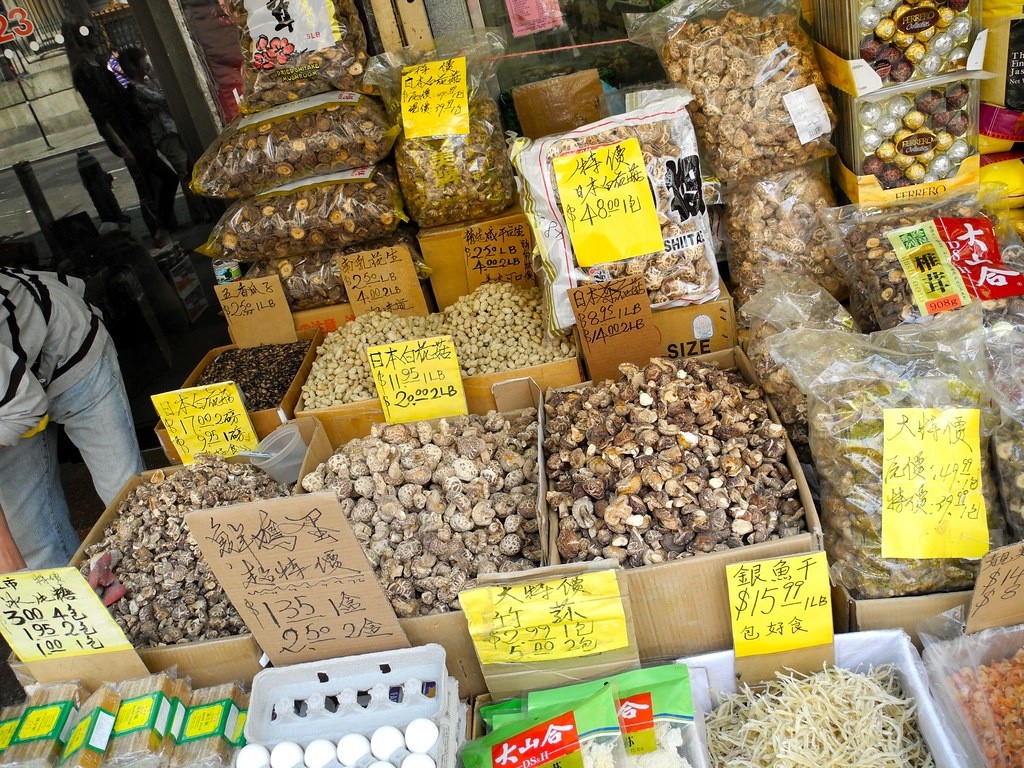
[69,0,1024,652]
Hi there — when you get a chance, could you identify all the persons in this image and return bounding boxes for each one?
[0,267,143,570]
[117,48,189,240]
[60,13,173,240]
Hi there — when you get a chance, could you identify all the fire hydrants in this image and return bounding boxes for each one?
[75,150,134,227]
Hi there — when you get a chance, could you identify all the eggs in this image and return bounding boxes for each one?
[235,719,437,768]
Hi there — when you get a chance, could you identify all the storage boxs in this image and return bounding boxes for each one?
[3,206,1024,768]
